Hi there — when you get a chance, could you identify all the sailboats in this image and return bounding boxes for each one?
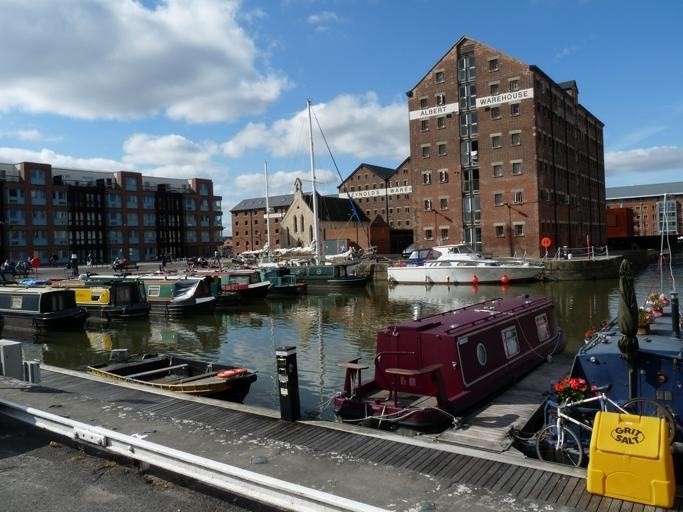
[237,95,377,287]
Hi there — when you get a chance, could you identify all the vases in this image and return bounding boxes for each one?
[636,327,649,335]
[653,307,662,317]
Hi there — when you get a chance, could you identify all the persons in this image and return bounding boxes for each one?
[1,257,30,280]
[112,257,122,273]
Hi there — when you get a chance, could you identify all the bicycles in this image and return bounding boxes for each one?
[535,384,675,470]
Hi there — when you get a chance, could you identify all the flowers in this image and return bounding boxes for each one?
[551,378,588,407]
[633,306,662,327]
[644,292,669,310]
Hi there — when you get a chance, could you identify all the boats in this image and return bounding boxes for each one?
[85,344,257,405]
[329,291,564,435]
[0,284,88,329]
[254,266,298,286]
[518,191,682,491]
[154,271,271,294]
[79,270,216,320]
[386,242,543,287]
[47,278,152,321]
